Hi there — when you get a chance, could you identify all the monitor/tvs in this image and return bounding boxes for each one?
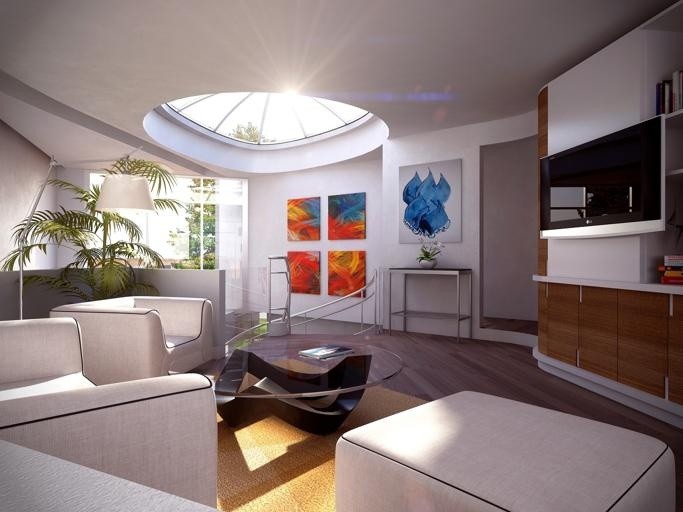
[537,113,665,239]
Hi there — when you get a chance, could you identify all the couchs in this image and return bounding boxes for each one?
[335,391,676,512]
[0,316,218,509]
[50,296,214,386]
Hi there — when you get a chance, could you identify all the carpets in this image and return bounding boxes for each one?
[206,358,429,512]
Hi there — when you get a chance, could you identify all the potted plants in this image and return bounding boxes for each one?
[415,236,445,269]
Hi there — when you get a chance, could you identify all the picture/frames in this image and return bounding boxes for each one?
[287,197,321,241]
[286,251,320,294]
[328,192,366,240]
[397,158,462,244]
[327,251,367,298]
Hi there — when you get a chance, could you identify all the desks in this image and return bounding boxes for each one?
[388,268,473,344]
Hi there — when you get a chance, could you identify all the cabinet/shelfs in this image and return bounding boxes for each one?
[532,3,683,432]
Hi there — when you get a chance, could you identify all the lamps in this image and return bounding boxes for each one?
[19,146,154,320]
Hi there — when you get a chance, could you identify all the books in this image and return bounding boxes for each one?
[656,70,683,115]
[659,254,683,285]
[298,343,355,362]
